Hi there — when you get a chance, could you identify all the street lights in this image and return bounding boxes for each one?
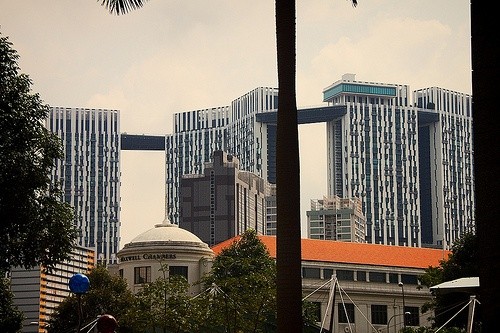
[386,311,411,333]
[398,282,407,332]
